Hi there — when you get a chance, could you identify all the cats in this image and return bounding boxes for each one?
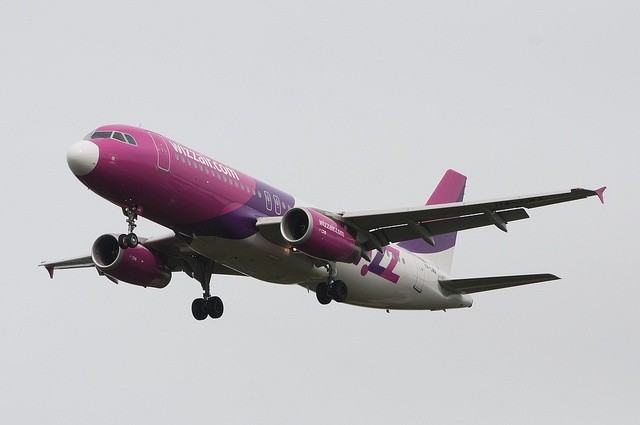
[38,124,607,320]
